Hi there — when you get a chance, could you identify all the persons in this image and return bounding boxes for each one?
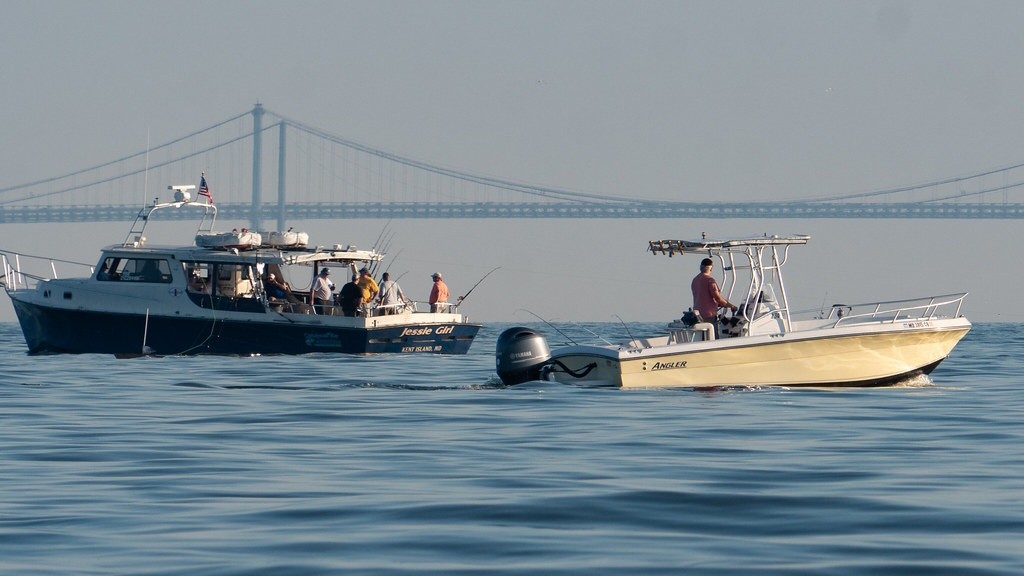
[97,263,111,281]
[692,259,737,341]
[428,272,452,313]
[264,268,408,318]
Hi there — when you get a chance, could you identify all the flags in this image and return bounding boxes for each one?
[198,176,213,204]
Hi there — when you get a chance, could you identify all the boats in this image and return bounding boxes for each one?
[494,232,972,388]
[0,183,486,356]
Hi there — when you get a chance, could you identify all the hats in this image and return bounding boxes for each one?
[321,267,331,275]
[430,272,441,278]
[358,268,369,274]
